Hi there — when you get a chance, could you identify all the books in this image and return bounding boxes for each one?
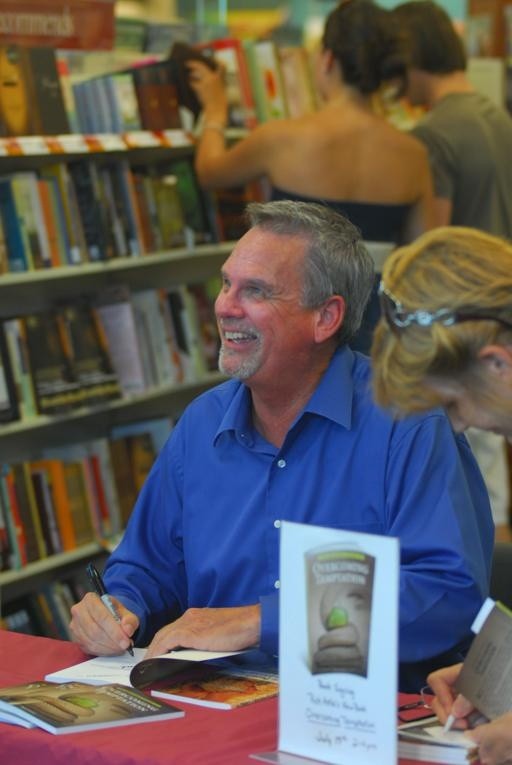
[71,61,181,131]
[370,83,430,130]
[0,276,221,425]
[0,555,109,641]
[452,601,512,721]
[198,38,331,129]
[0,418,175,576]
[397,717,480,765]
[43,647,257,690]
[0,156,215,274]
[150,673,279,711]
[216,179,271,242]
[0,680,185,737]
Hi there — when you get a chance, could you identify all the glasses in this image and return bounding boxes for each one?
[377,280,510,337]
[397,684,437,725]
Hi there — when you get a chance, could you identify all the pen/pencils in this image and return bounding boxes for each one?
[85,561,141,660]
[437,708,463,736]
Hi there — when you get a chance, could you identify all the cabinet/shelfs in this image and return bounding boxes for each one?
[0,57,506,632]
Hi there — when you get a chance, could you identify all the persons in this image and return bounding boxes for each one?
[67,199,496,695]
[387,0,512,243]
[369,226,512,764]
[182,1,450,359]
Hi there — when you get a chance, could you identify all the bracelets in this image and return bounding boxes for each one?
[200,121,226,136]
